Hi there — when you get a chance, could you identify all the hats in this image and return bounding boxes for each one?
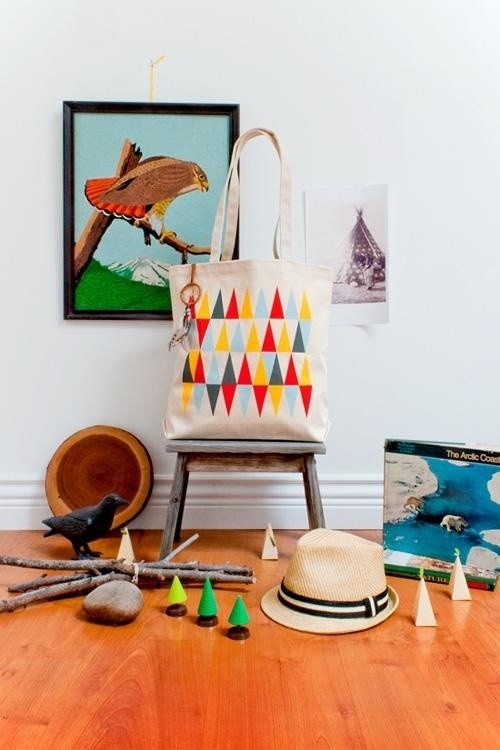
[259,527,401,636]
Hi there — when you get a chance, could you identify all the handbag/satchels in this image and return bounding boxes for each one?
[165,123,338,444]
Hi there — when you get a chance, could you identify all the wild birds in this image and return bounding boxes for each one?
[41,494,129,560]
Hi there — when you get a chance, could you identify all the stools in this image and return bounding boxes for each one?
[159,436,327,563]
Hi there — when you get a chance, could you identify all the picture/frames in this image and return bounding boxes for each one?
[59,96,239,325]
